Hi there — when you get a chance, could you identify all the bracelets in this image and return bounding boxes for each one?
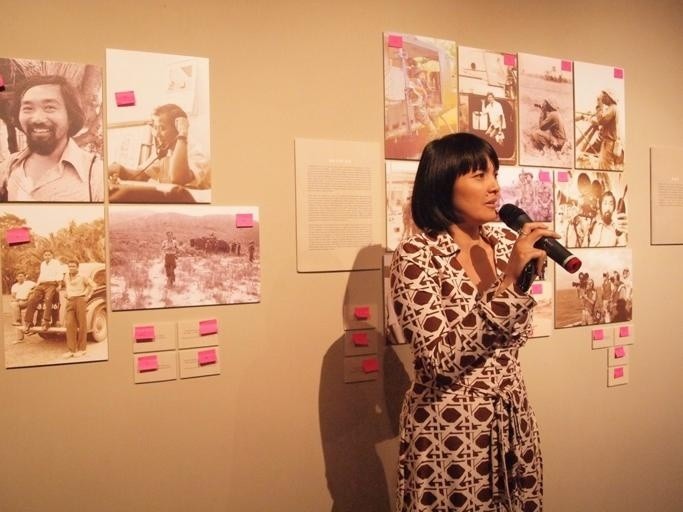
[176,135,188,142]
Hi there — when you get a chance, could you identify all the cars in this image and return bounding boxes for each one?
[17,262,107,343]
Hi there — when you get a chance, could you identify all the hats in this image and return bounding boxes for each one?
[603,90,620,105]
[545,97,560,110]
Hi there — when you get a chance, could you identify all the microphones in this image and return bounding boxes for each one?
[496,204,581,275]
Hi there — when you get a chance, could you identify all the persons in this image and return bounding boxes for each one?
[11,250,97,358]
[527,98,564,159]
[564,174,629,248]
[190,235,255,262]
[107,103,211,190]
[574,270,632,325]
[589,89,619,171]
[407,65,446,136]
[0,74,104,202]
[518,171,555,223]
[161,230,179,285]
[481,93,506,145]
[389,132,562,512]
